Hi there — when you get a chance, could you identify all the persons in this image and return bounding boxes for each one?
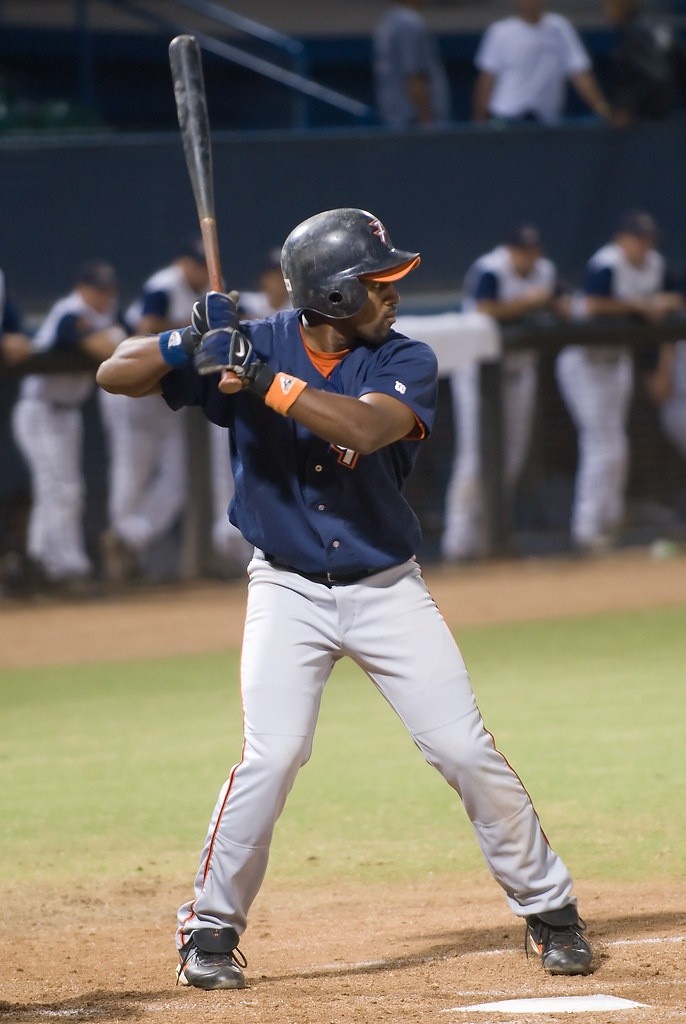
[0,0,686,594]
[97,208,594,991]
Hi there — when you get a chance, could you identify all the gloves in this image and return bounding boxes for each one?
[191,326,276,403]
[158,291,242,371]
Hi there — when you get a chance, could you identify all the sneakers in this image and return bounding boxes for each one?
[175,929,247,989]
[524,904,591,975]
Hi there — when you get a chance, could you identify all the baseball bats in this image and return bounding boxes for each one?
[166,32,244,392]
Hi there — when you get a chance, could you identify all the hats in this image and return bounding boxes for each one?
[619,211,660,239]
[182,231,209,261]
[511,222,543,249]
[359,256,420,284]
[79,260,117,292]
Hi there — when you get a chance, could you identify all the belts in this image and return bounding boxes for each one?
[263,553,378,585]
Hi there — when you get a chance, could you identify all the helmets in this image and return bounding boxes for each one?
[280,205,422,323]
